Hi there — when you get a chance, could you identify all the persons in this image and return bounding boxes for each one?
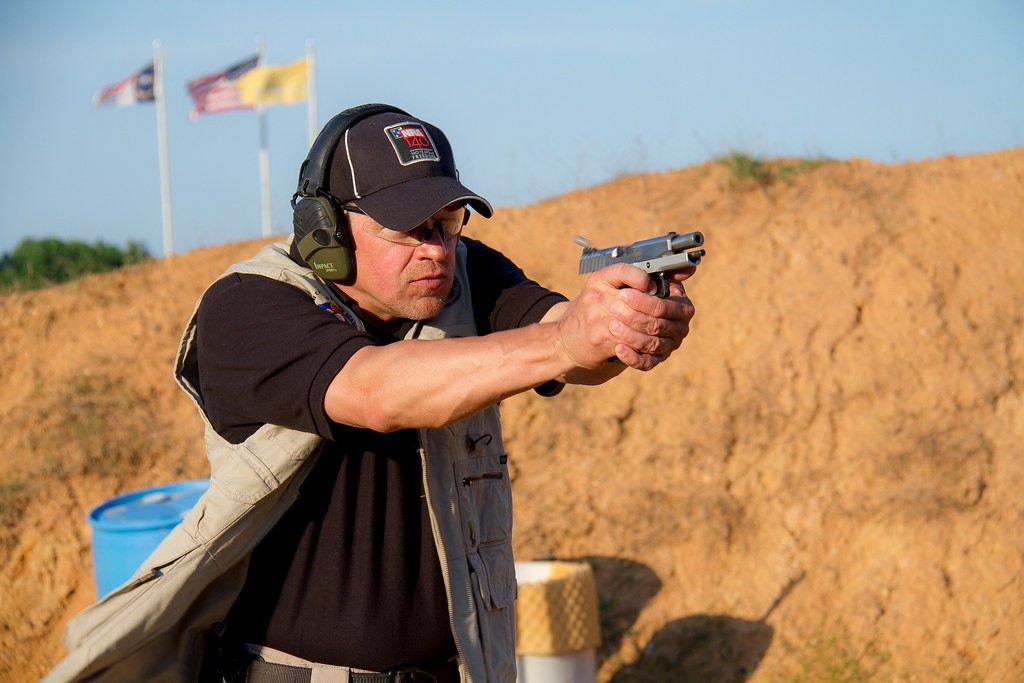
[40,103,695,683]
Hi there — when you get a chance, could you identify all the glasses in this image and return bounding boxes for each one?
[337,205,466,245]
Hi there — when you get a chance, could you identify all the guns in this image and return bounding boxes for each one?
[578,230,707,297]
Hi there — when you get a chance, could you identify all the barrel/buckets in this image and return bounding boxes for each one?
[89,480,211,601]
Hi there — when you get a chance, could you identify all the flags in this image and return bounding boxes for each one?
[187,57,258,117]
[93,64,154,106]
[240,60,306,103]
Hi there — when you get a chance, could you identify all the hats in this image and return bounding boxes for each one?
[301,113,493,231]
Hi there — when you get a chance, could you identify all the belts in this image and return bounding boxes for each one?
[209,646,460,683]
